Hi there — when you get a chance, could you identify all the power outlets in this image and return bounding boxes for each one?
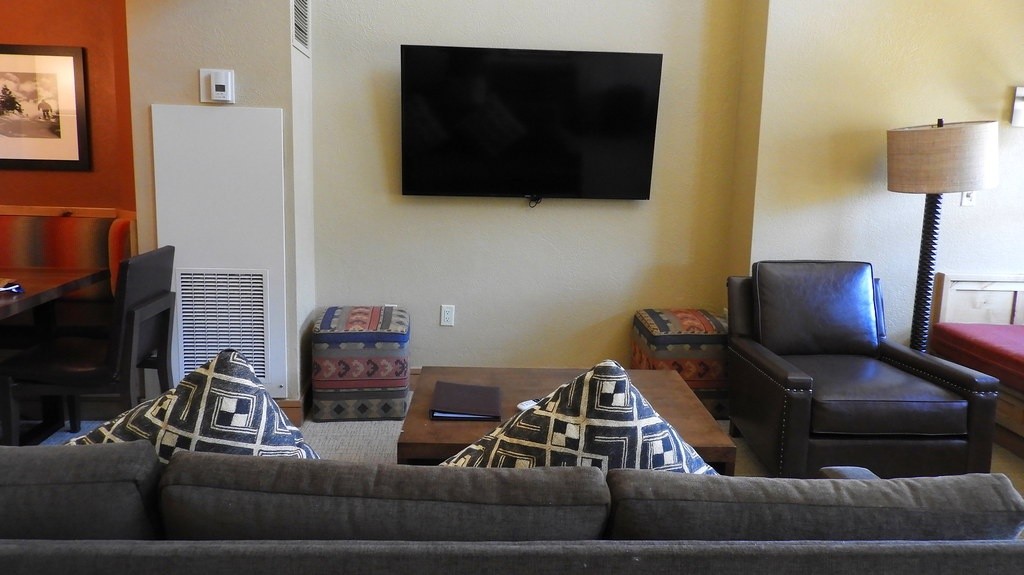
[440,305,455,326]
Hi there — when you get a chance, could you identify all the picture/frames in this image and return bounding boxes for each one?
[0,44,91,171]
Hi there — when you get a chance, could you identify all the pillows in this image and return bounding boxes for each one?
[420,359,733,478]
[65,351,326,459]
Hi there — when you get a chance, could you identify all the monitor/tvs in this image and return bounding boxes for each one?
[400,44,664,201]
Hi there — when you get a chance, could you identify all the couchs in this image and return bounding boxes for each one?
[728,259,998,475]
[0,443,1024,575]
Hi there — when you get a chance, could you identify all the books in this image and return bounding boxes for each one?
[427,379,503,421]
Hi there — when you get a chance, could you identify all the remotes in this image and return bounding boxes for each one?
[517,399,544,410]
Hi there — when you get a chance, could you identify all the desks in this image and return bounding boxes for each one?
[397,367,738,478]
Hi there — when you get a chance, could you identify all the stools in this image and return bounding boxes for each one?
[312,305,410,418]
[629,307,728,411]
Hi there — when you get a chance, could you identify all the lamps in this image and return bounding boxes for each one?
[886,114,999,359]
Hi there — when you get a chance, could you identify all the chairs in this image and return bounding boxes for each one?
[58,246,178,432]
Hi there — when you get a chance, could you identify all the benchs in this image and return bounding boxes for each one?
[0,205,138,301]
